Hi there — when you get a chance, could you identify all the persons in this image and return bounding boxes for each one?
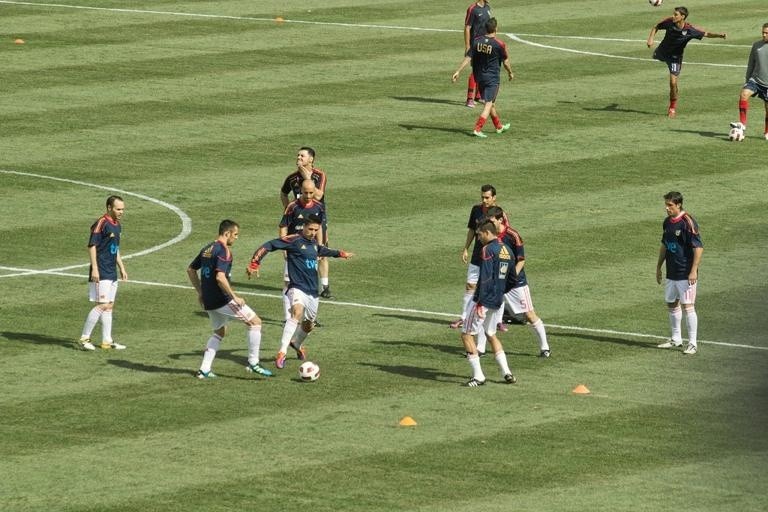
[280,147,333,298]
[656,191,704,355]
[730,23,768,140]
[78,195,126,350]
[187,220,272,377]
[245,213,353,369]
[647,7,726,118]
[461,185,552,386]
[279,179,328,328]
[464,0,491,108]
[452,17,514,138]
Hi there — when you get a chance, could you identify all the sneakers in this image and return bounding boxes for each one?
[196,370,217,379]
[275,352,286,369]
[472,122,510,138]
[729,122,746,131]
[467,97,485,109]
[290,341,306,360]
[450,319,507,358]
[668,107,675,119]
[78,338,96,351]
[464,379,483,386]
[318,285,337,301]
[100,340,127,349]
[540,345,552,358]
[657,338,683,348]
[245,362,273,377]
[505,374,516,383]
[683,343,697,354]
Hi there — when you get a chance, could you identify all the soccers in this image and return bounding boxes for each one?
[299,362,320,381]
[649,0,663,7]
[729,128,744,142]
